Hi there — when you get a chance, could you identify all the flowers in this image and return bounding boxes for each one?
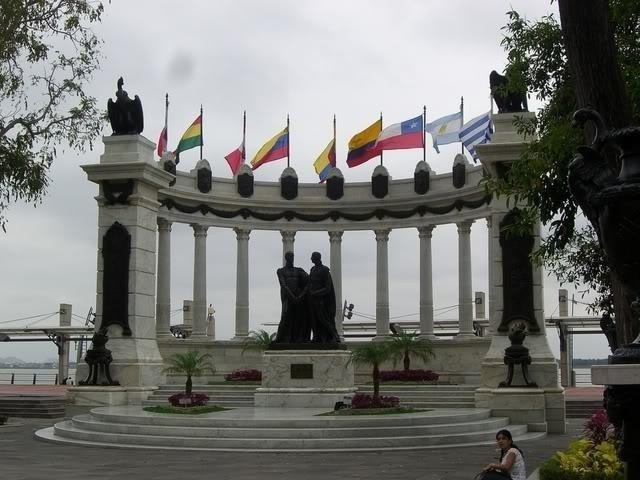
[352,394,399,408]
[377,370,438,380]
[224,369,262,381]
[168,393,209,407]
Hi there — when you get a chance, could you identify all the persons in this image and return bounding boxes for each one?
[482,429,527,480]
[308,252,341,343]
[276,252,311,343]
[62,378,68,384]
[66,376,73,385]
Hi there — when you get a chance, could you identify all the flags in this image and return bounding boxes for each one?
[425,112,463,154]
[249,127,289,171]
[313,139,336,183]
[459,111,493,163]
[372,114,424,154]
[173,116,203,164]
[346,119,381,168]
[158,125,168,157]
[225,129,246,175]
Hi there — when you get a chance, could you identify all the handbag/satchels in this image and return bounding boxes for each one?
[480,466,512,479]
